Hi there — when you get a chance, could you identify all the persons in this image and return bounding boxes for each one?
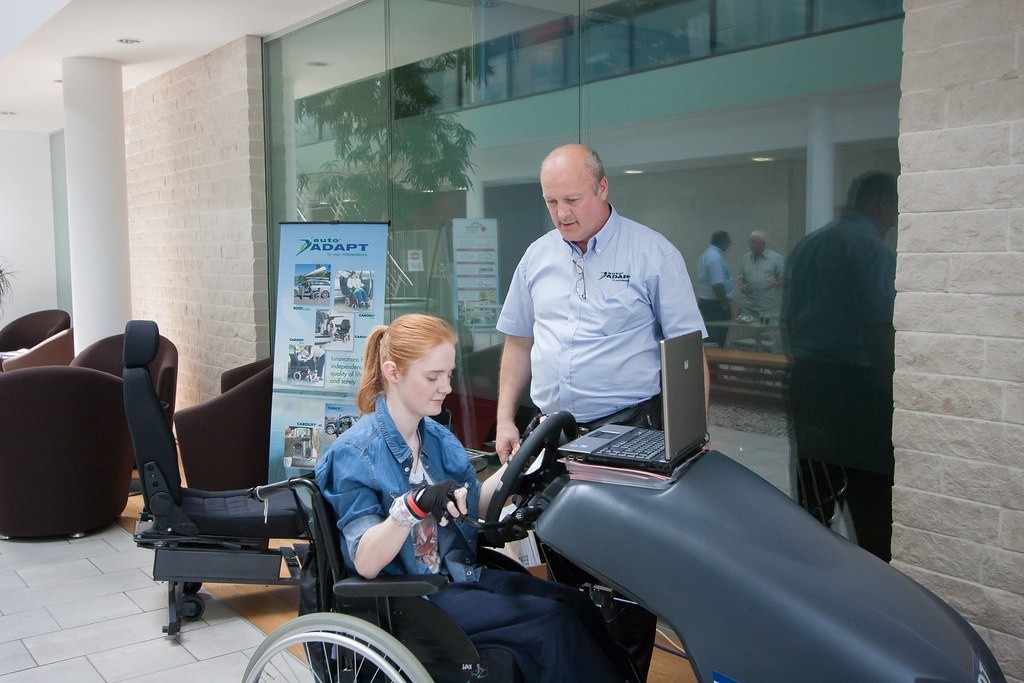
[297,345,326,380]
[317,313,640,683]
[734,229,789,385]
[697,230,737,379]
[346,271,370,306]
[782,170,898,565]
[494,143,709,683]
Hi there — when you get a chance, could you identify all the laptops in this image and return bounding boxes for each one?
[556,330,710,478]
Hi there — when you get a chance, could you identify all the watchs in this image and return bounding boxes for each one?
[706,431,710,442]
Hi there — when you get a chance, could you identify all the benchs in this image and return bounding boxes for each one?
[702,345,790,400]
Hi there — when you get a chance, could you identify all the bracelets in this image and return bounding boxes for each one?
[399,490,427,524]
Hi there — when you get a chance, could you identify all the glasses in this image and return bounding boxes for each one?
[572,257,586,298]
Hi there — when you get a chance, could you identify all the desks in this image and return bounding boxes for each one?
[704,320,784,383]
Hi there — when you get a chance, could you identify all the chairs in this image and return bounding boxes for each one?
[122,320,311,637]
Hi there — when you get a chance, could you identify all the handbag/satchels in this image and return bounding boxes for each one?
[577,395,661,432]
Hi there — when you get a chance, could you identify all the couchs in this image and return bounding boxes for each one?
[0,309,74,373]
[0,365,136,539]
[70,333,178,470]
[174,357,275,492]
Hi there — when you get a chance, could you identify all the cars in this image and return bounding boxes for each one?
[294,278,330,299]
[324,415,360,436]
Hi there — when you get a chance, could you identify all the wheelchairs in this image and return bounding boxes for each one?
[339,276,371,310]
[292,352,321,383]
[241,477,657,683]
